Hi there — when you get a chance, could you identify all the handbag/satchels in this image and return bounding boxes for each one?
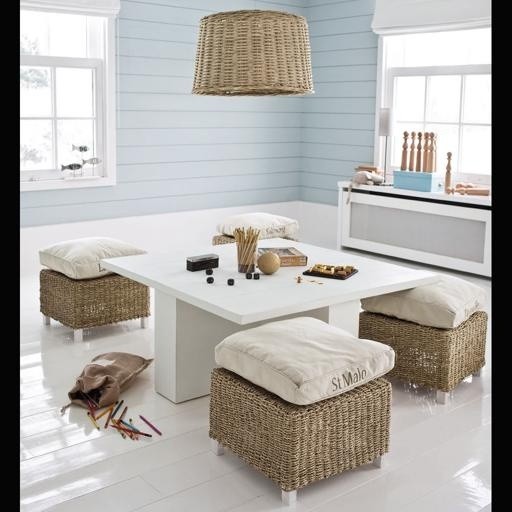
[61,352,154,416]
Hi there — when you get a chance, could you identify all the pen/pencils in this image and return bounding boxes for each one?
[233,226,260,273]
[80,390,161,440]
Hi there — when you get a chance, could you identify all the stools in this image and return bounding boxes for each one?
[209,368,393,506]
[40,270,150,342]
[358,312,488,403]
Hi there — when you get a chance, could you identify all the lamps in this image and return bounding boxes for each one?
[379,107,393,185]
[193,10,314,97]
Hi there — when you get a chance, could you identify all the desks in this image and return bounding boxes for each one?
[98,237,446,403]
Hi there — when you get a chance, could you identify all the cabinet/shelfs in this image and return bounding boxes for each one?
[337,182,492,278]
